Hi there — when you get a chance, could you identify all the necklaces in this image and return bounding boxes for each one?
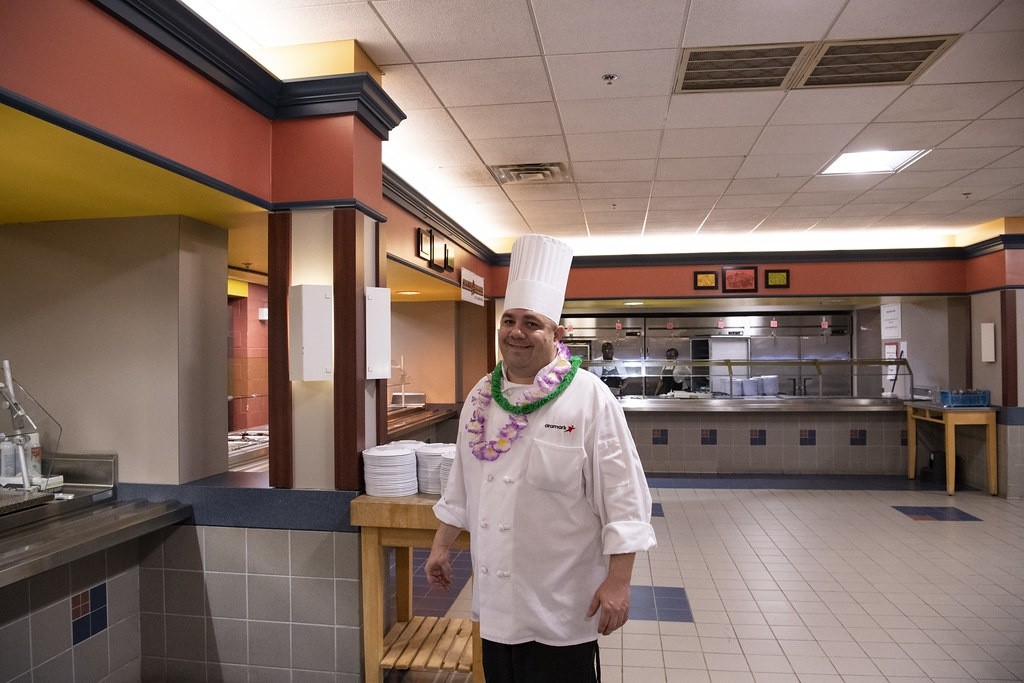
[464,342,583,461]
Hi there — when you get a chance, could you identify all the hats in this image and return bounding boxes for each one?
[503,234,574,327]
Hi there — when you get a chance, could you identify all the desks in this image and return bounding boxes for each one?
[903,402,999,496]
[350,492,485,683]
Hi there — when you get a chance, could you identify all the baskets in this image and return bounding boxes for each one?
[939,387,990,408]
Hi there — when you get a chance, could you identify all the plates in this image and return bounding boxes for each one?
[363,440,424,498]
[416,443,456,497]
[721,376,779,395]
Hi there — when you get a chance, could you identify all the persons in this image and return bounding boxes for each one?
[588,342,629,396]
[655,348,690,396]
[425,234,658,683]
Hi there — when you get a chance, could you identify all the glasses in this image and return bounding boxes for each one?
[603,348,614,352]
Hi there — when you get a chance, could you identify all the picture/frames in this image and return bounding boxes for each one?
[722,266,758,293]
[694,271,718,290]
[765,269,789,288]
[461,267,484,307]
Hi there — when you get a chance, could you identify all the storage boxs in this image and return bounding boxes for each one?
[939,390,990,407]
